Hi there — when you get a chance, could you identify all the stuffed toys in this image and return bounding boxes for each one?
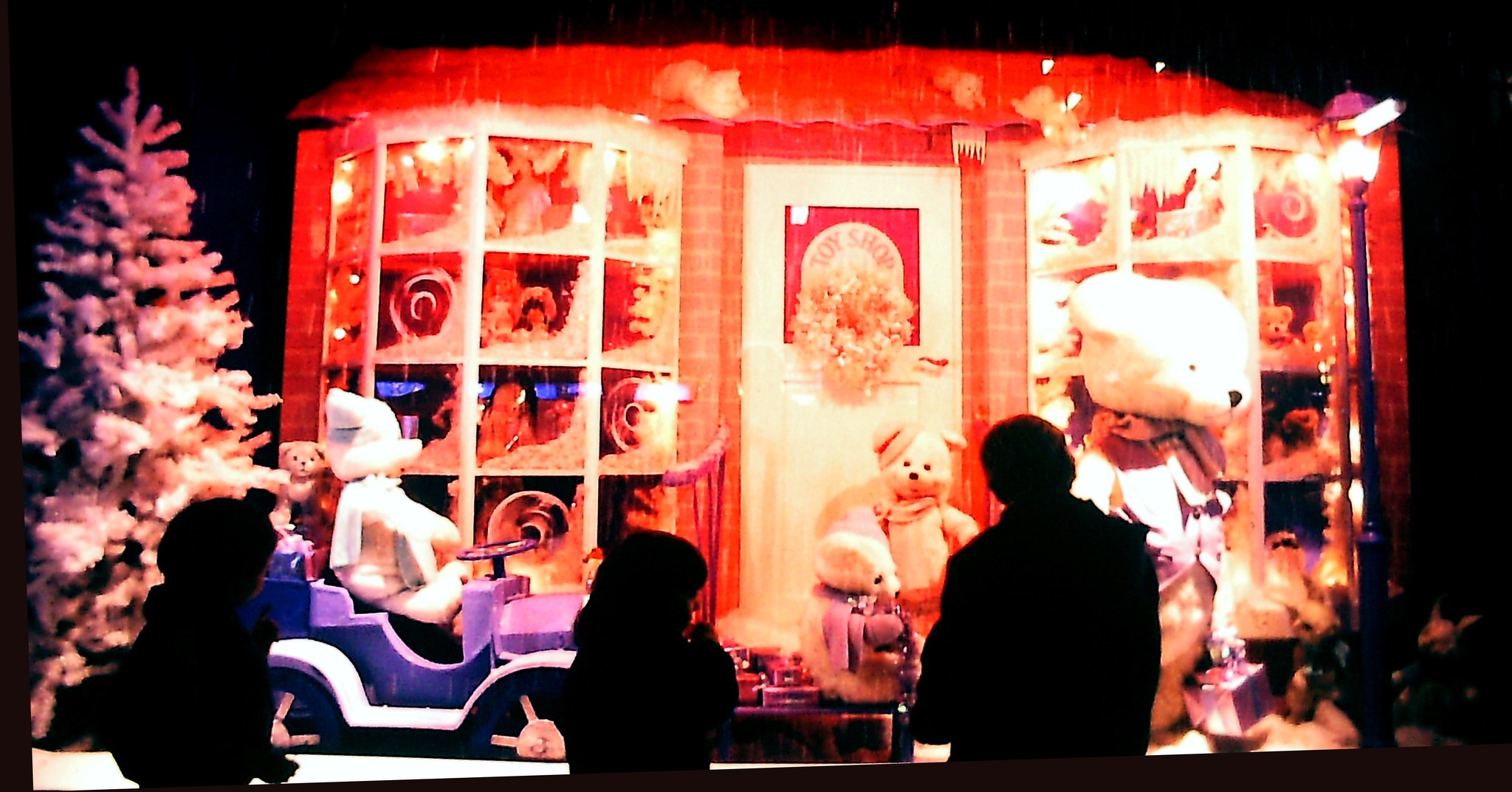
[865,409,981,641]
[319,388,468,625]
[270,439,328,536]
[1059,274,1257,739]
[800,530,925,704]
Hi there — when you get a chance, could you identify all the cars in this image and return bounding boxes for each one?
[240,533,589,761]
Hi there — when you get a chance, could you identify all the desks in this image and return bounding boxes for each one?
[712,707,895,764]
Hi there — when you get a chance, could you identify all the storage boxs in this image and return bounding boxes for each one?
[1184,661,1273,738]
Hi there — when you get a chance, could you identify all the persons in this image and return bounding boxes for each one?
[104,495,300,792]
[896,412,1161,760]
[569,530,740,791]
[1231,570,1512,751]
